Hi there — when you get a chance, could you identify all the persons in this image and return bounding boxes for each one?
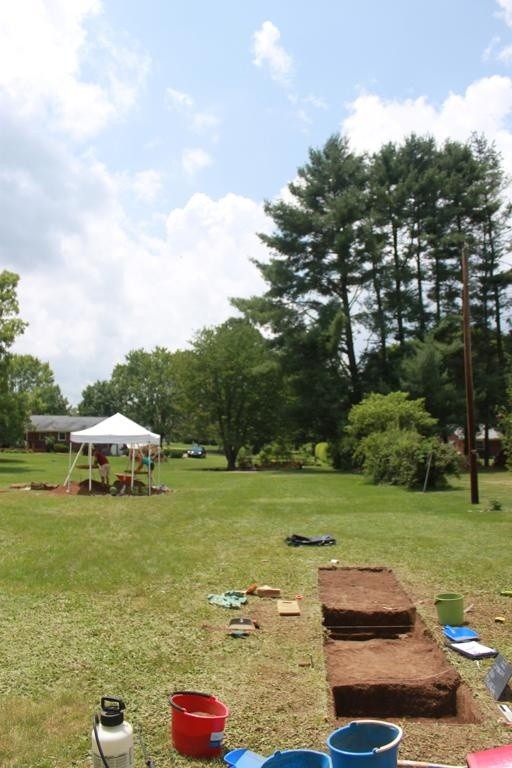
[134,455,156,486]
[91,449,111,484]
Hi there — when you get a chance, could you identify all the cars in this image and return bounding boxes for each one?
[187,446,206,458]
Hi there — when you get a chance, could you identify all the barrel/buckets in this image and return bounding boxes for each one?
[328,720,403,766]
[262,748,330,766]
[167,691,229,759]
[435,592,466,628]
